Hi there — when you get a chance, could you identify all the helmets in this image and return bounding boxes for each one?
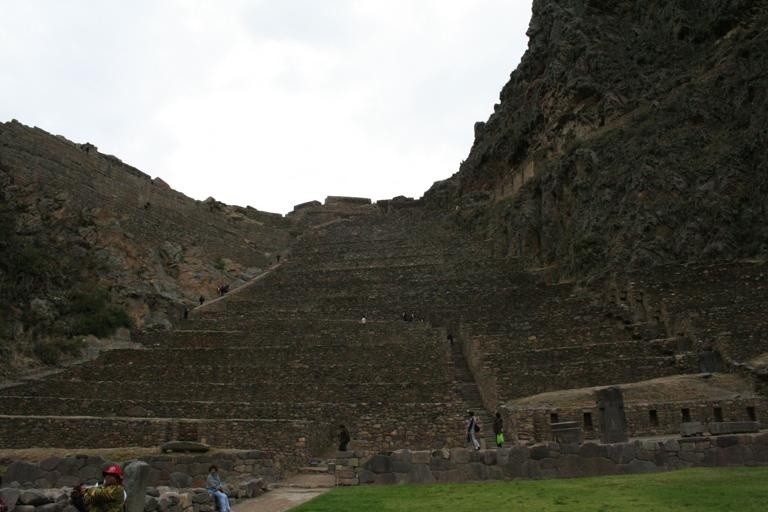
[102,465,124,483]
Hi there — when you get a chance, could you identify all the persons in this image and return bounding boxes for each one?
[467,412,480,451]
[199,295,203,305]
[276,255,280,261]
[79,465,125,512]
[447,335,453,346]
[338,424,350,451]
[206,464,230,512]
[184,308,188,319]
[493,412,505,449]
[220,286,224,296]
[361,316,366,324]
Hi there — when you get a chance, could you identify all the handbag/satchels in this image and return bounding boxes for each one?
[475,424,480,432]
[467,437,471,442]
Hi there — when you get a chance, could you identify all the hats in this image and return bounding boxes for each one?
[209,465,218,472]
